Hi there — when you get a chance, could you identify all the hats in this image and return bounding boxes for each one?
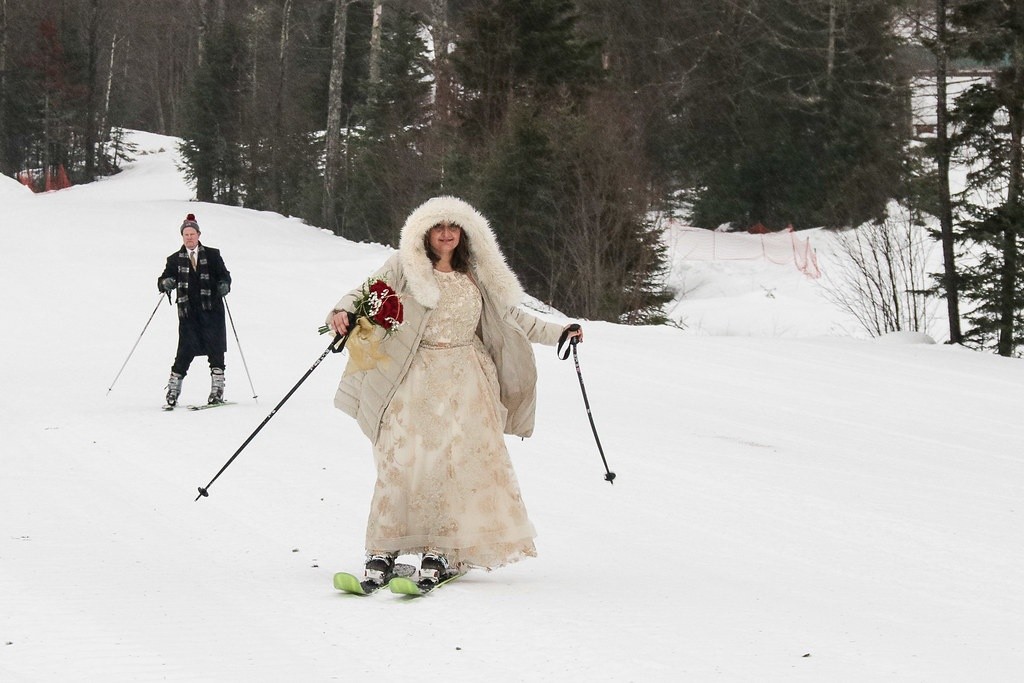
[180,214,199,235]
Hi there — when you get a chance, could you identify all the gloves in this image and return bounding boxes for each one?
[163,278,176,291]
[219,283,229,296]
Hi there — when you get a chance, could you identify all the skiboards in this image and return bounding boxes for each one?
[333,558,470,597]
[162,399,231,412]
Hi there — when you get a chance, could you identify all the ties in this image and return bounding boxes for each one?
[190,251,196,272]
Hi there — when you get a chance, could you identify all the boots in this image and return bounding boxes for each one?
[208,367,225,404]
[166,366,188,406]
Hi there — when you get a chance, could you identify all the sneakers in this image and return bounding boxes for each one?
[418,551,449,587]
[364,552,395,584]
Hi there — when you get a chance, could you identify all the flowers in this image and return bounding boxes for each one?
[319,275,410,342]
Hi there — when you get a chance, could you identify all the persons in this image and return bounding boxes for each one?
[325,196,583,584]
[157,213,232,411]
